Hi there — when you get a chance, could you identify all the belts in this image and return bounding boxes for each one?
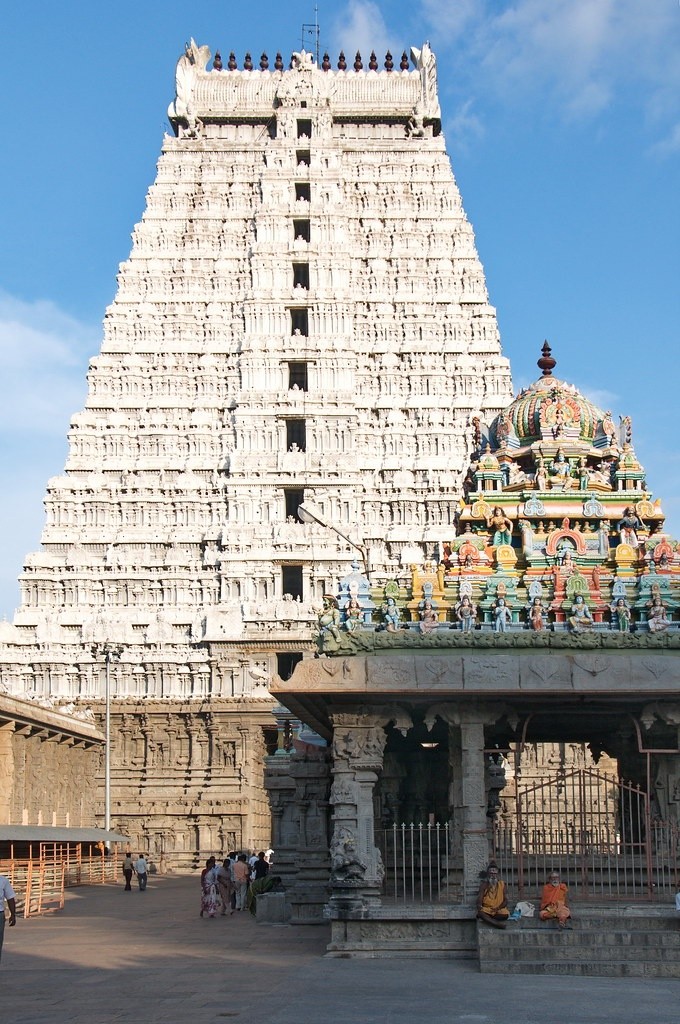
[0,911,4,913]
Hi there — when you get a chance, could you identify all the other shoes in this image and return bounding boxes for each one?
[231,910,234,915]
[209,915,215,917]
[221,913,228,916]
[200,909,203,916]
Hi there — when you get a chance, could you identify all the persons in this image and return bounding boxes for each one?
[551,400,569,443]
[675,882,680,914]
[135,854,148,891]
[531,446,592,493]
[616,507,643,548]
[179,101,204,142]
[199,849,269,919]
[122,852,135,891]
[463,552,672,574]
[539,870,573,929]
[475,860,510,929]
[484,505,514,546]
[0,874,16,960]
[408,103,427,140]
[309,580,669,643]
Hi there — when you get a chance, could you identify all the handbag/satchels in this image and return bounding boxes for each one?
[514,901,535,917]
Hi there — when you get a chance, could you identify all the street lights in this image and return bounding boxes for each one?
[297,500,372,583]
[91,637,123,880]
